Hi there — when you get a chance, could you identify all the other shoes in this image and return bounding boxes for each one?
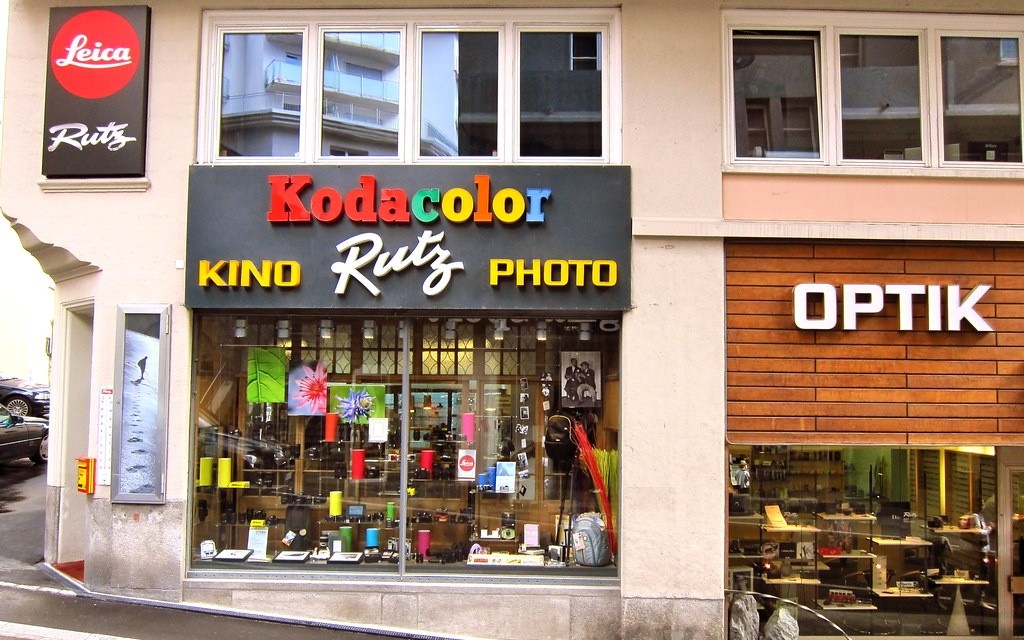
[578,400,598,407]
[567,395,577,402]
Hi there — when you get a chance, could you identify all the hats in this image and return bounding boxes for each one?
[580,361,589,366]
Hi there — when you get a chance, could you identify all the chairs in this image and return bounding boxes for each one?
[189,428,1023,638]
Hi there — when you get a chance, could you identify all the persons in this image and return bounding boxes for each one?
[970,500,991,605]
[576,361,596,406]
[924,515,952,607]
[735,459,750,494]
[515,372,553,468]
[563,358,581,401]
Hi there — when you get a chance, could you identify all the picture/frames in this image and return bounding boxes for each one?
[109,301,173,507]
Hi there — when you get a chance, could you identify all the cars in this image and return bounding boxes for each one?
[0,377,49,418]
[0,403,48,467]
